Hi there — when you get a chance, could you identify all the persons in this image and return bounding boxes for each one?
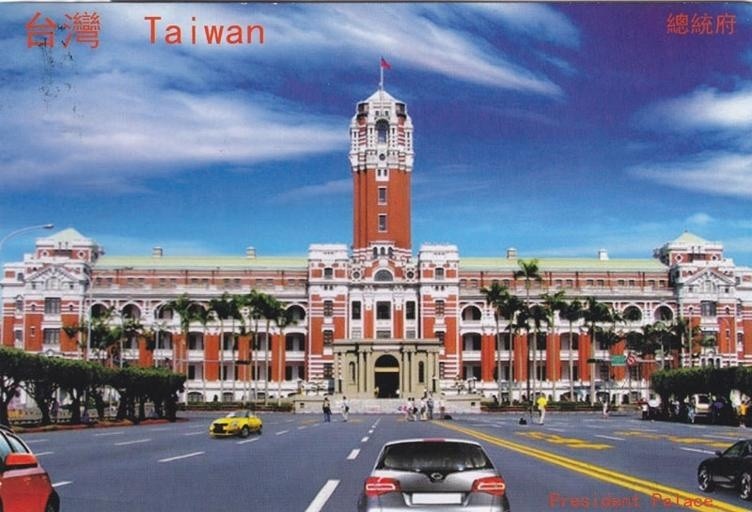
[423,386,427,398]
[393,396,445,421]
[602,400,609,419]
[634,396,697,424]
[321,396,331,423]
[340,396,349,422]
[708,397,752,428]
[52,398,59,423]
[374,383,380,397]
[536,395,547,425]
[395,387,400,398]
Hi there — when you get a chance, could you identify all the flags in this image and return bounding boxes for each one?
[380,55,392,70]
